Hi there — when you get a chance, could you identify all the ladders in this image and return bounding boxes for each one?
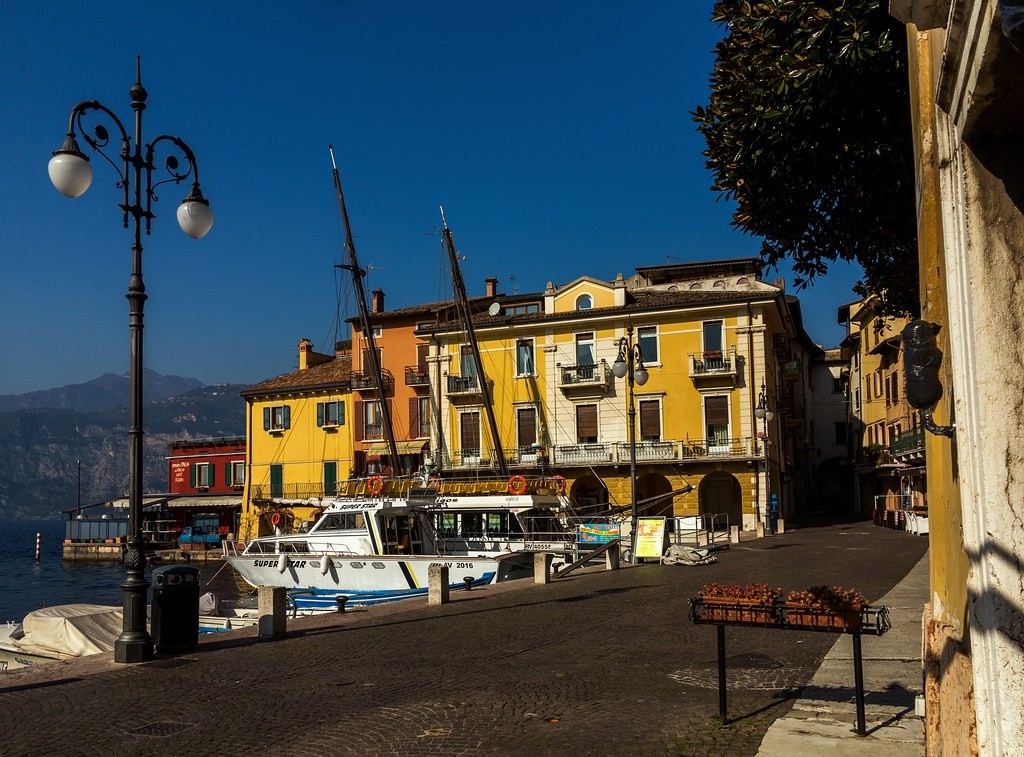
[414,510,453,556]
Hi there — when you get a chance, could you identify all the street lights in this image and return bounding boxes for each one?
[754,374,774,537]
[48,54,215,661]
[612,311,649,564]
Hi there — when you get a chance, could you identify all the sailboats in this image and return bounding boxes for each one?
[308,202,579,561]
[219,144,589,589]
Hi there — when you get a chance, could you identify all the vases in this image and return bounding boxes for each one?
[786,603,862,627]
[702,595,774,624]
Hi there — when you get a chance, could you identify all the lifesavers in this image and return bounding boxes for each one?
[368,476,383,494]
[428,476,440,491]
[549,476,566,494]
[271,513,281,525]
[509,474,527,495]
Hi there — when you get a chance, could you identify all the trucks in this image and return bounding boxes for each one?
[178,526,220,547]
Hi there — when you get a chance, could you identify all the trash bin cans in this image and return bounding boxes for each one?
[152,565,200,649]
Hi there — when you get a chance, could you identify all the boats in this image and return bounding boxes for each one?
[200,573,497,638]
[0,603,257,670]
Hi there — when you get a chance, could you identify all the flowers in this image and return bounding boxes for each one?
[787,585,869,608]
[697,582,784,601]
